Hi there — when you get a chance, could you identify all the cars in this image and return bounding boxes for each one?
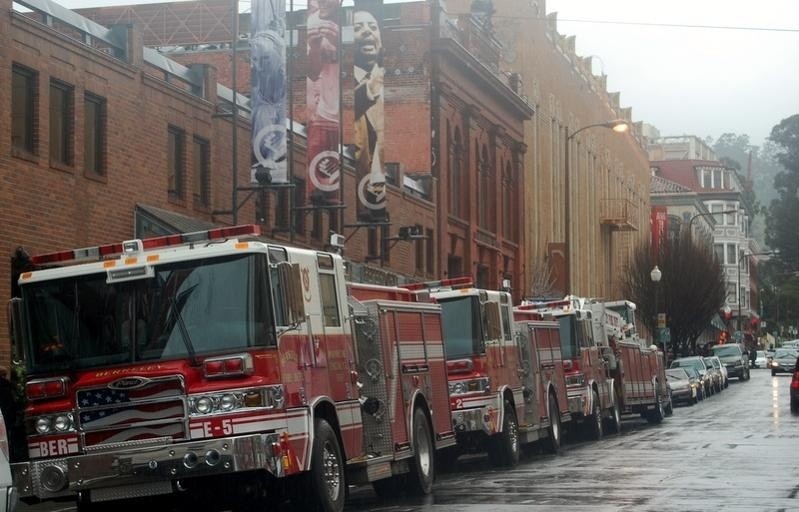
[0,408,18,512]
[663,336,798,415]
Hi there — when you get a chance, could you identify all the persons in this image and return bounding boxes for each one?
[353,11,385,216]
[742,347,748,355]
[750,347,757,369]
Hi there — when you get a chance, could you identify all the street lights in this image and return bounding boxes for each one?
[562,117,634,294]
[651,262,664,350]
[736,248,782,332]
[722,305,733,342]
[689,208,739,243]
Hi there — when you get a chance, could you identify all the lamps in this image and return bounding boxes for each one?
[208,166,422,264]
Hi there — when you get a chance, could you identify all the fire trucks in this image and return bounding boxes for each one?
[0,222,460,512]
[387,271,576,470]
[516,288,675,439]
